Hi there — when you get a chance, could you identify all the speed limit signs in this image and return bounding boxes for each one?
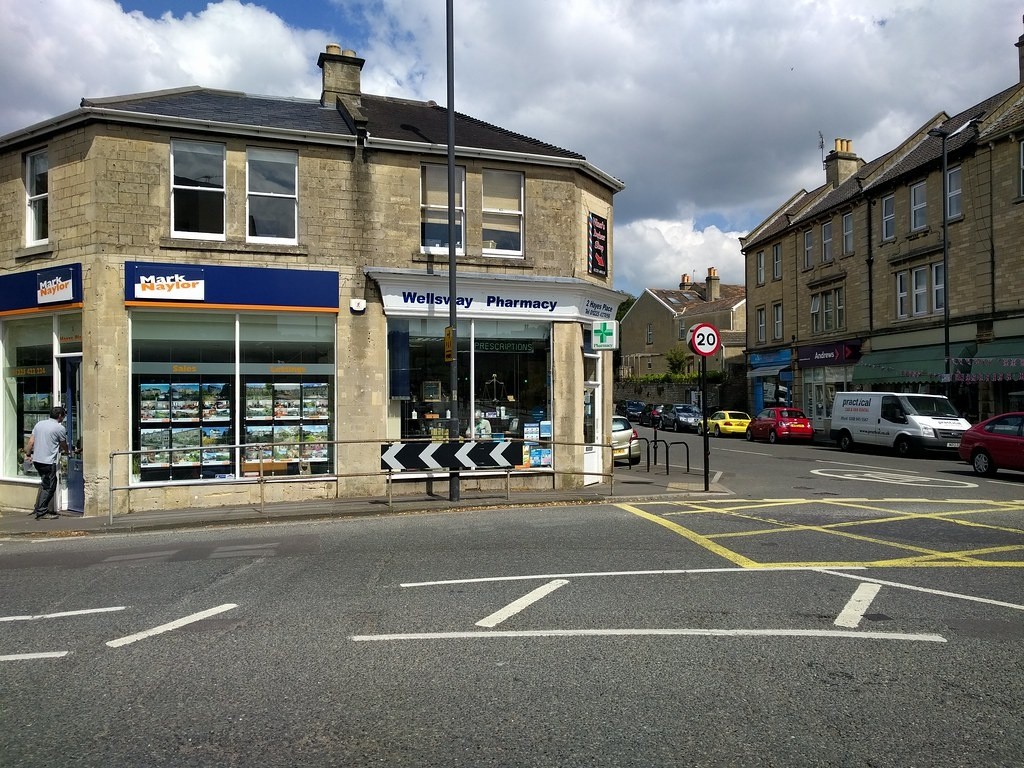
[692,323,721,356]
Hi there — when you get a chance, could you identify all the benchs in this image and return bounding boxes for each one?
[490,424,511,432]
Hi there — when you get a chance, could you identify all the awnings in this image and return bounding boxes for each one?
[747,365,789,377]
[853,343,976,385]
[971,339,1024,382]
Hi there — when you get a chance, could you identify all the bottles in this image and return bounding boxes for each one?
[412,409,417,419]
[446,409,451,418]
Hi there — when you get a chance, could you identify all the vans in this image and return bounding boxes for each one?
[829,392,972,459]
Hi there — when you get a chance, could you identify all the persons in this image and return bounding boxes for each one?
[465,409,491,438]
[26,407,67,519]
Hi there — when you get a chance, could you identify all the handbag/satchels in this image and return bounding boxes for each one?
[23,457,34,472]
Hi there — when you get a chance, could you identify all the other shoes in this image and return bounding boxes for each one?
[36,514,59,521]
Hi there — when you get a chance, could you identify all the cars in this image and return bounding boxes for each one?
[625,402,647,422]
[611,414,641,466]
[698,409,751,439]
[638,403,664,428]
[616,400,635,417]
[746,407,814,445]
[658,403,703,433]
[958,411,1024,478]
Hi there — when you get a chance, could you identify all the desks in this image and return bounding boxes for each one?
[407,418,451,436]
[475,416,517,436]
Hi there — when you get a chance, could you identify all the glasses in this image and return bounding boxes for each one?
[59,413,67,418]
